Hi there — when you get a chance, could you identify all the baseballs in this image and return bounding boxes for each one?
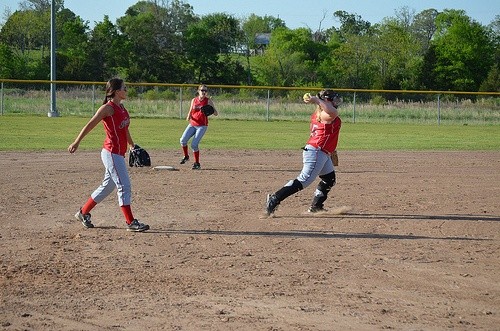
[303,93,311,99]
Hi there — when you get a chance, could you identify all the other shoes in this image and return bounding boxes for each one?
[180,155,189,164]
[192,162,201,169]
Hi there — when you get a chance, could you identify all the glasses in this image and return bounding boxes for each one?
[201,89,207,92]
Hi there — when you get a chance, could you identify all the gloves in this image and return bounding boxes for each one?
[300,146,308,151]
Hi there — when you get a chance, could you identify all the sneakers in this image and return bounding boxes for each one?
[266,193,280,214]
[125,219,150,232]
[307,206,328,213]
[74,208,94,228]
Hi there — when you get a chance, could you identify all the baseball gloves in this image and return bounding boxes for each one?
[128,144,151,167]
[331,151,338,166]
[200,105,214,116]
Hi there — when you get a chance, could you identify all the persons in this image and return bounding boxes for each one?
[180,85,218,170]
[266,90,342,217]
[67,77,151,232]
